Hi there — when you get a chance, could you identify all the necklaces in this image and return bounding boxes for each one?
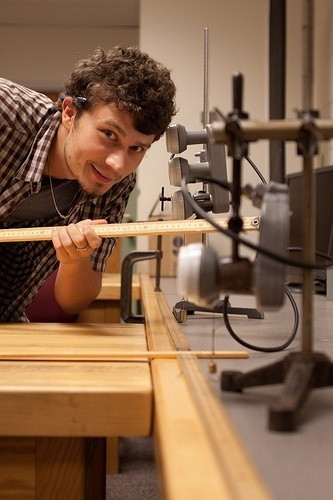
[48,156,83,218]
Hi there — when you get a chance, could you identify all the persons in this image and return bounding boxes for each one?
[0,45,177,325]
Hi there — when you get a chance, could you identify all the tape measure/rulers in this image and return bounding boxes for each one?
[0,215,262,243]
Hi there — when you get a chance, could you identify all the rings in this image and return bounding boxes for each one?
[75,244,90,251]
